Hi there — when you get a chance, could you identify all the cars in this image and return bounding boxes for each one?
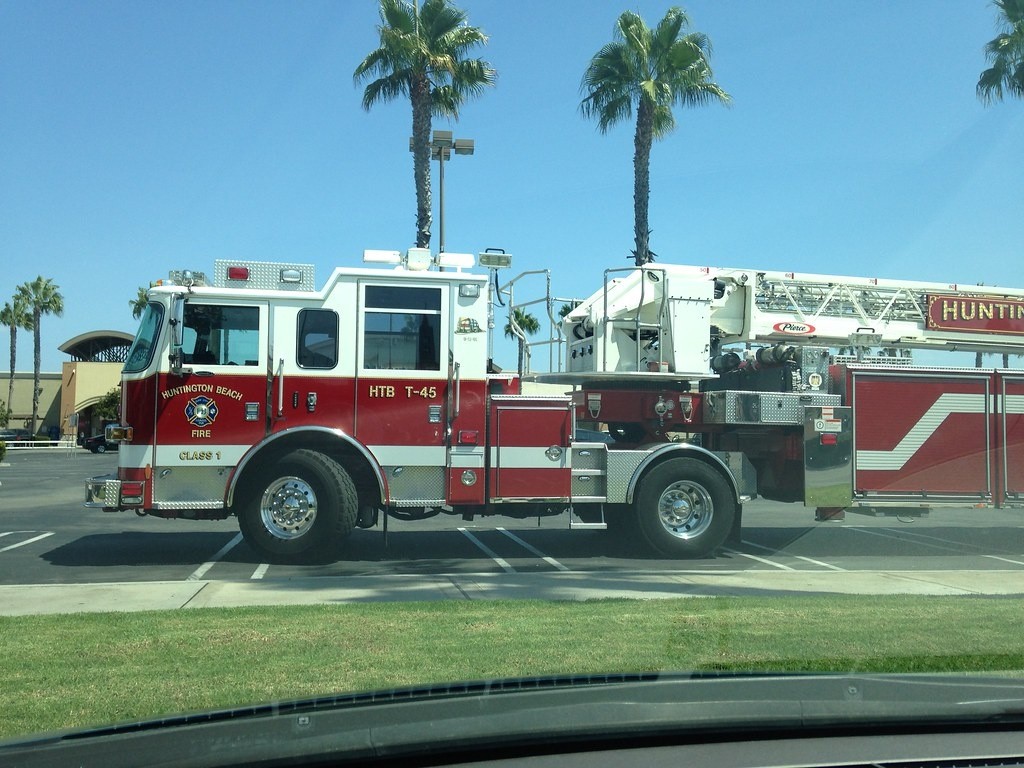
[0,428,32,447]
[82,433,118,454]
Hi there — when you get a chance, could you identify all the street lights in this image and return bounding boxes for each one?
[409,127,474,270]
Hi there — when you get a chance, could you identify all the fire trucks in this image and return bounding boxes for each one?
[84,246,1024,566]
